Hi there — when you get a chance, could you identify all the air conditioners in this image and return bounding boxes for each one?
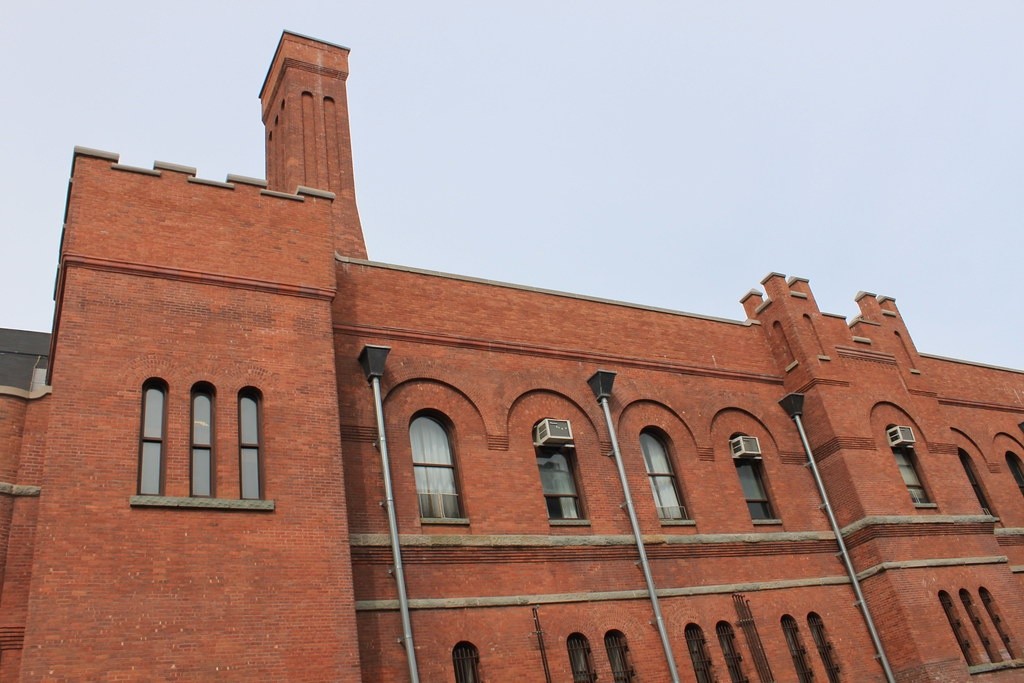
[731,436,762,457]
[536,418,574,444]
[886,426,916,445]
